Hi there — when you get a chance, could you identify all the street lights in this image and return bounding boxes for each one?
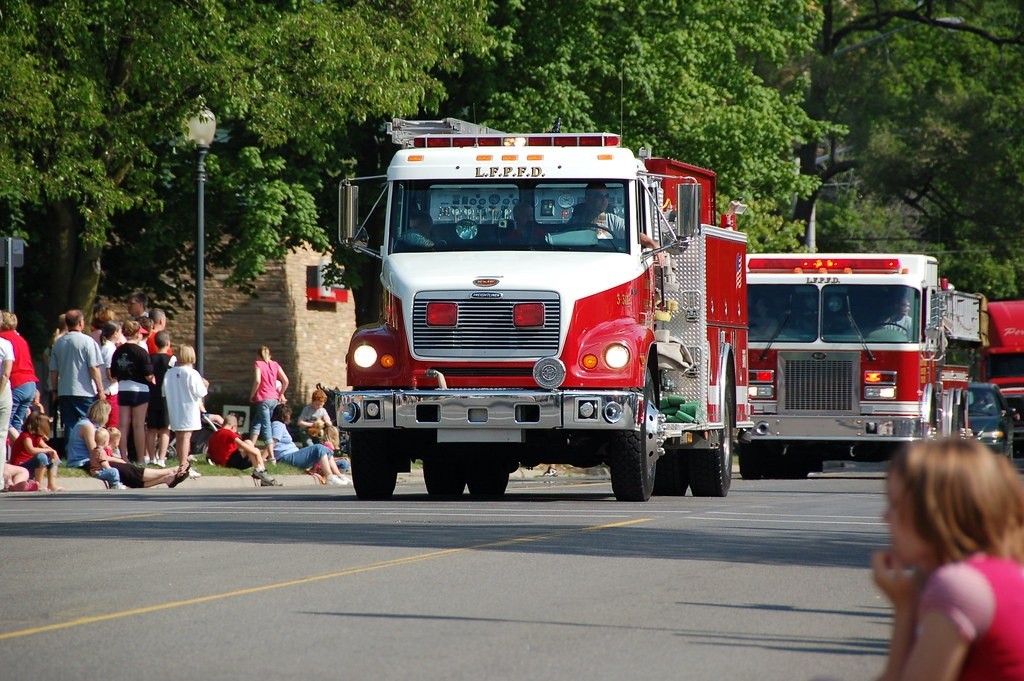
[182,95,216,384]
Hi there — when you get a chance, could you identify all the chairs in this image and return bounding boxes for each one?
[572,203,615,217]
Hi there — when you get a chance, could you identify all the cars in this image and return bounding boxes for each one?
[963,383,1016,463]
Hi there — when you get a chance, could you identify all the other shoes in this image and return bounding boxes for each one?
[268,457,277,468]
[326,474,353,486]
[111,482,127,490]
[8,481,39,491]
[46,486,64,492]
[157,459,167,467]
[144,455,158,465]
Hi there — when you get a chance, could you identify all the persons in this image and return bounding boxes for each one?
[161,344,209,478]
[208,415,283,486]
[8,413,63,492]
[298,390,332,448]
[0,310,44,492]
[66,399,192,488]
[567,183,666,269]
[249,345,289,465]
[89,428,128,490]
[104,427,121,456]
[47,292,177,468]
[200,402,224,425]
[880,295,912,335]
[873,437,1024,681]
[261,404,353,486]
[275,380,287,403]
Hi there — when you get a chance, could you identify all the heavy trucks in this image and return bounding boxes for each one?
[978,300,1024,447]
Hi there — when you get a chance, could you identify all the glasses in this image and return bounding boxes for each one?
[895,302,910,307]
[586,192,609,199]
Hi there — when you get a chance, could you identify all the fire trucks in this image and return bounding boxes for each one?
[332,117,754,503]
[739,252,988,482]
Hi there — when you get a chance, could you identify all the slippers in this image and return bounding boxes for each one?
[167,463,191,488]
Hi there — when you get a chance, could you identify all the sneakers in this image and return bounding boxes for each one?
[261,477,282,486]
[252,468,275,482]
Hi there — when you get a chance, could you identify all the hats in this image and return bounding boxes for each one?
[122,325,148,337]
